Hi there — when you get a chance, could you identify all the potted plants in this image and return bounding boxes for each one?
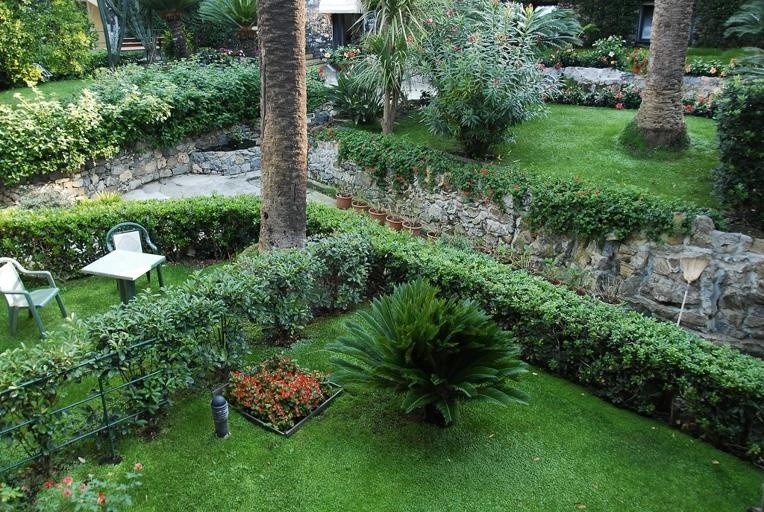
[335,187,626,306]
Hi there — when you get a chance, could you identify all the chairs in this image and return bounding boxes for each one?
[0,257,68,338]
[106,222,164,290]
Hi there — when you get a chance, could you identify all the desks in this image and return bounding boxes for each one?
[80,250,166,303]
